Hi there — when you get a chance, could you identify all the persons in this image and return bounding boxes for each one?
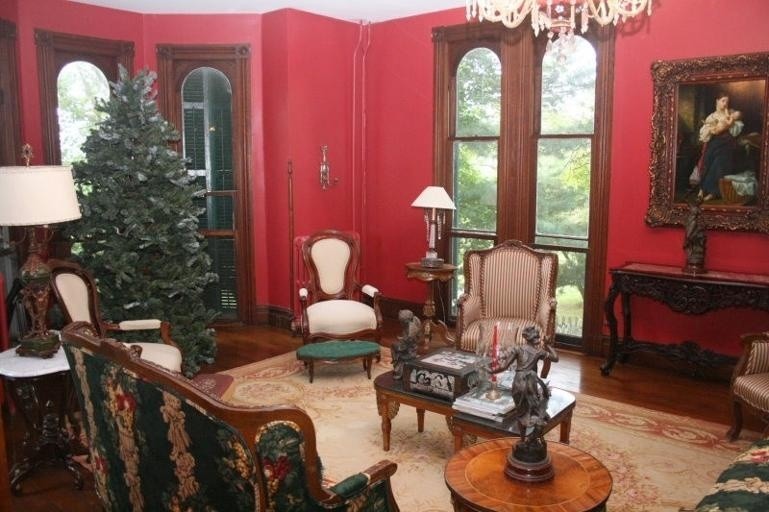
[479,324,559,455]
[686,91,746,204]
[678,182,709,268]
[387,308,428,379]
[699,108,743,143]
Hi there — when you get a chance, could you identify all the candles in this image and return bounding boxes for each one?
[490,323,499,382]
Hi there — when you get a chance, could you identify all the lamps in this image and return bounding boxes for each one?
[467,1,652,62]
[0,146,82,357]
[411,185,457,268]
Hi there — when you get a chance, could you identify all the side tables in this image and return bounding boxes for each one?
[445,438,613,511]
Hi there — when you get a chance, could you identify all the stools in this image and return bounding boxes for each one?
[296,340,380,383]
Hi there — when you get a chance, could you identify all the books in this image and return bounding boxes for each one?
[451,369,552,426]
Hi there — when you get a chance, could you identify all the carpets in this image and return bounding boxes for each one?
[212,340,766,511]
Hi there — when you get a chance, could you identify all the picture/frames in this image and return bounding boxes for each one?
[645,52,769,235]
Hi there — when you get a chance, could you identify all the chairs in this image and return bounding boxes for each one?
[299,229,382,367]
[693,443,769,511]
[458,239,558,378]
[47,260,183,377]
[726,332,768,443]
[62,321,402,512]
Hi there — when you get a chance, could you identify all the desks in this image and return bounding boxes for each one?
[600,261,768,378]
[405,262,455,356]
[0,344,84,494]
